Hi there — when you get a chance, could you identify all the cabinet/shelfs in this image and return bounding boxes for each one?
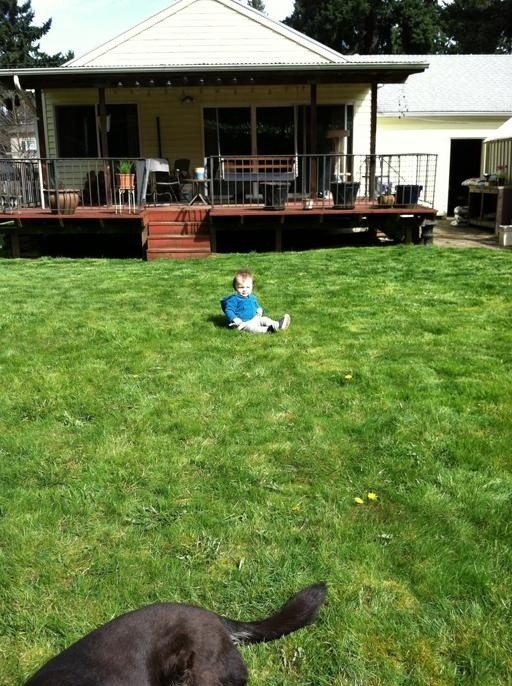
[466,185,512,234]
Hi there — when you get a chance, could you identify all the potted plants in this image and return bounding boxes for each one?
[117,157,135,189]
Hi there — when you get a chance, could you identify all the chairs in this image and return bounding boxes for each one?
[147,153,219,206]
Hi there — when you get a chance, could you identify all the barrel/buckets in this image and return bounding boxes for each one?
[193,167,205,179]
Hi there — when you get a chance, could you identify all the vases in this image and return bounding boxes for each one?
[378,195,394,207]
[43,189,80,215]
[302,199,314,210]
[498,179,505,185]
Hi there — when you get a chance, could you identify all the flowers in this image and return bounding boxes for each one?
[496,164,507,179]
[382,183,393,195]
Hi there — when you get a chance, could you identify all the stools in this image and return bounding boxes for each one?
[115,189,135,214]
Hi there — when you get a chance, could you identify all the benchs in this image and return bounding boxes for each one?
[220,156,295,199]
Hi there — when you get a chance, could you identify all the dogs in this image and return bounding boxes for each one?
[20,580,329,686]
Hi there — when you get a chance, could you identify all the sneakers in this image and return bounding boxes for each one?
[280,314,291,329]
[268,324,278,333]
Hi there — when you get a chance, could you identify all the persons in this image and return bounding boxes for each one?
[219,270,290,333]
